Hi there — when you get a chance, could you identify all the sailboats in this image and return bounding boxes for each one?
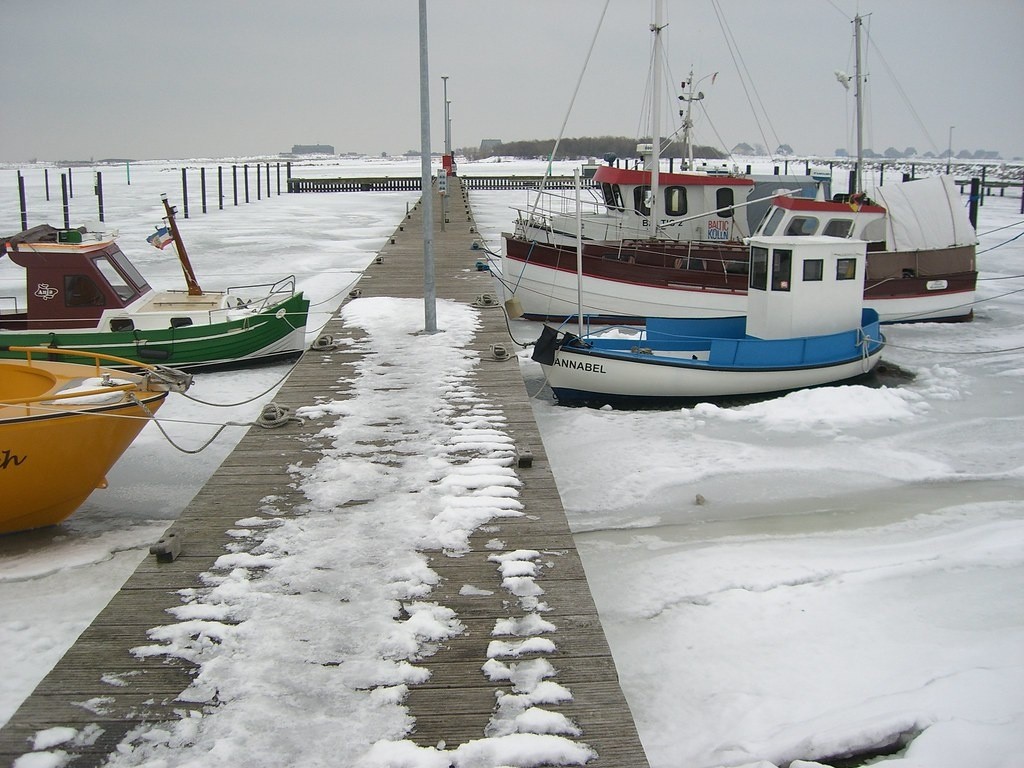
[495,0,979,326]
[511,0,820,253]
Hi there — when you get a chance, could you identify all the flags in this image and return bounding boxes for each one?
[147,227,174,251]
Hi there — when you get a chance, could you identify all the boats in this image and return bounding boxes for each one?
[0,343,195,536]
[533,235,886,400]
[0,190,310,378]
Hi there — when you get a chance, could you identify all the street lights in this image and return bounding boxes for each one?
[445,100,452,155]
[440,76,450,155]
